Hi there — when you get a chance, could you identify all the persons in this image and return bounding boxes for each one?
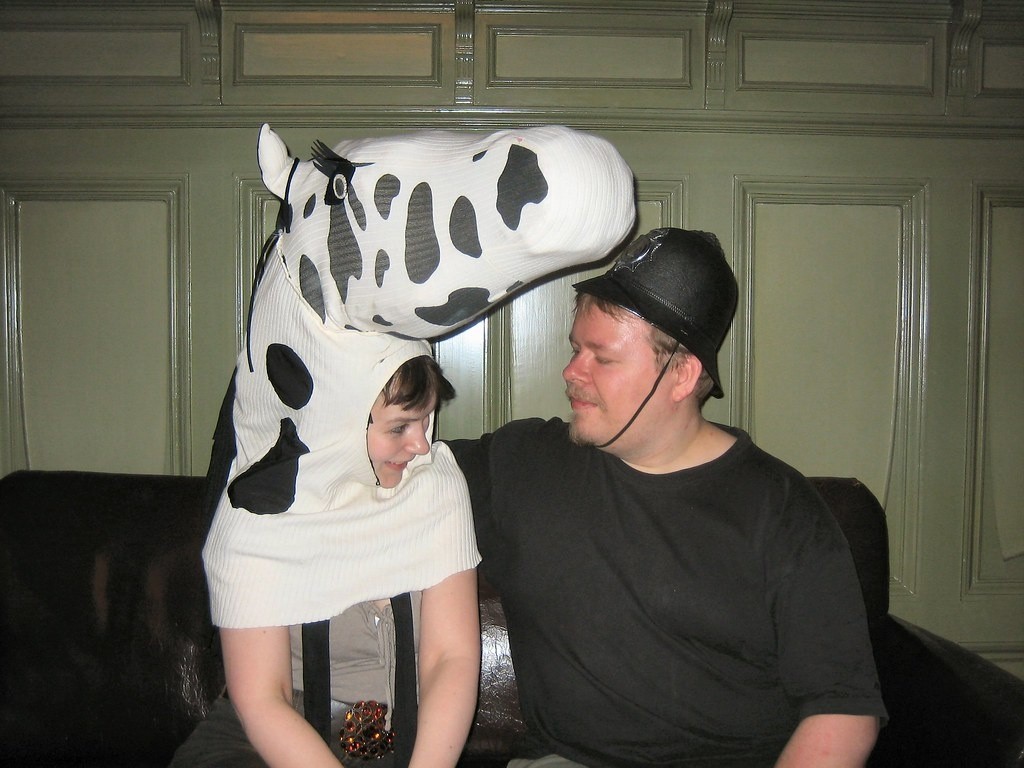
[170,342,484,767]
[443,228,889,768]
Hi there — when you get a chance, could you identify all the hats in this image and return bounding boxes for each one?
[571,227,739,399]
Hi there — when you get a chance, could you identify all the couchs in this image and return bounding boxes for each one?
[0,469,1022,768]
